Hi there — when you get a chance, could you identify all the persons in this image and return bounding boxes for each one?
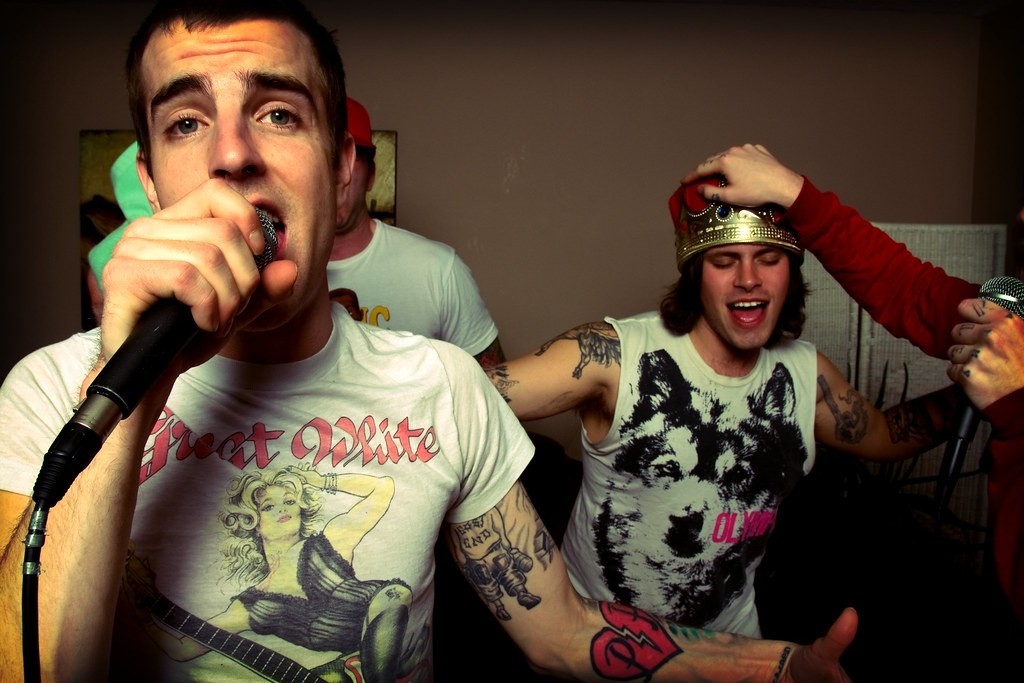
[486,173,972,683]
[81,95,506,373]
[694,142,1024,630]
[0,0,858,683]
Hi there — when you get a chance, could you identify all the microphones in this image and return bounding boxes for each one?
[938,276,1024,504]
[32,209,279,508]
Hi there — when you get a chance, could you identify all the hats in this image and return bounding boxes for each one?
[346,93,377,147]
[667,166,805,273]
